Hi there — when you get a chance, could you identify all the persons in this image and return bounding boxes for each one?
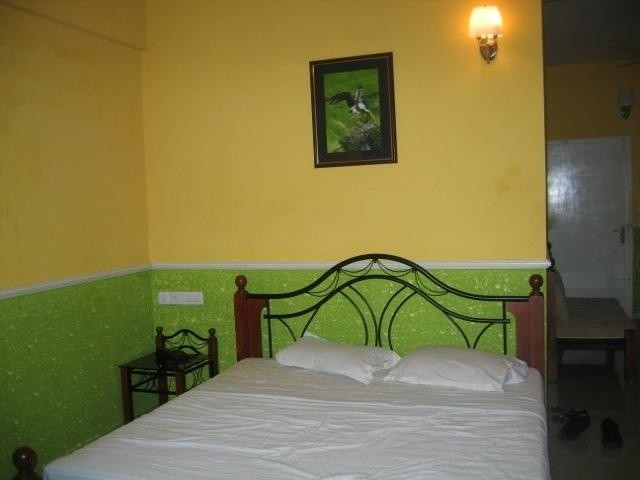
[355,87,373,118]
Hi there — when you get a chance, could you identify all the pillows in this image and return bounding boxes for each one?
[385,344,529,392]
[276,329,401,384]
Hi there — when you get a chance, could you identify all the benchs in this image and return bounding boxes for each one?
[547,266,635,387]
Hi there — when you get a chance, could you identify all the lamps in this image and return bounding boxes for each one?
[619,87,637,119]
[467,6,504,64]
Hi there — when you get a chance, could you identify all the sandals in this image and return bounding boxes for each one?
[599,416,623,449]
[557,410,591,441]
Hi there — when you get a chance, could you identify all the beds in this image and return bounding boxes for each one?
[11,252,549,480]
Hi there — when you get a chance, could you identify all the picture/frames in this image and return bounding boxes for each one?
[309,51,397,166]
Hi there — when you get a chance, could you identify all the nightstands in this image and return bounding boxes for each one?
[119,326,220,425]
